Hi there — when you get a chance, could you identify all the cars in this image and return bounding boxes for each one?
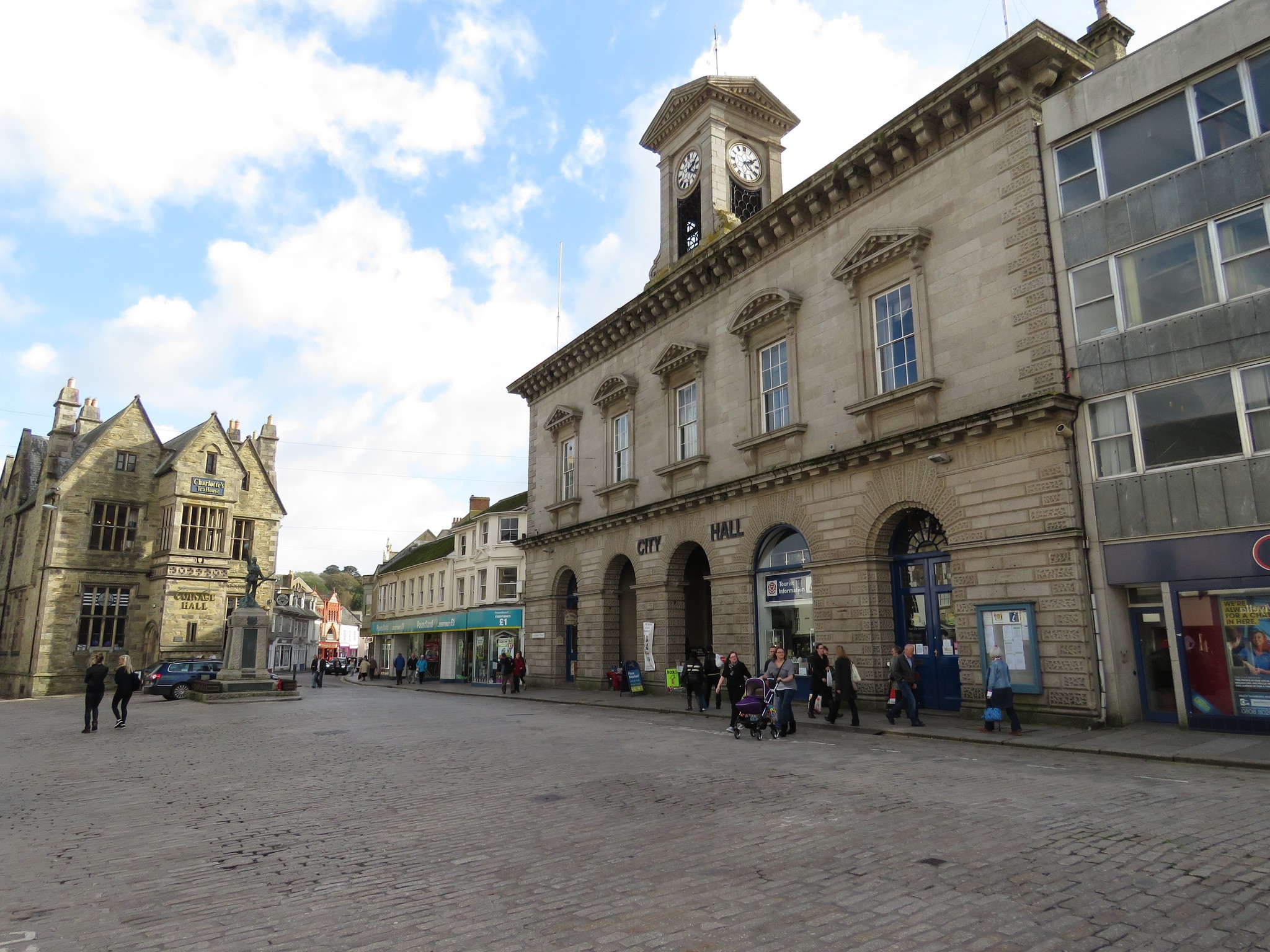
[270,673,280,680]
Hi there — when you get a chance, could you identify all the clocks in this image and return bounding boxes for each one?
[725,135,767,188]
[672,142,702,200]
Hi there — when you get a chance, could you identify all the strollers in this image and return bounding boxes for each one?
[732,677,779,740]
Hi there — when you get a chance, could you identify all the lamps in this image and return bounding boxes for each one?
[541,548,555,554]
[926,452,951,466]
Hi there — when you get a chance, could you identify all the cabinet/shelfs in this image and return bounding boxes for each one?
[785,628,796,660]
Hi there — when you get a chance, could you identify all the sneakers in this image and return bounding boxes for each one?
[114,719,126,729]
[726,727,735,733]
[737,724,745,730]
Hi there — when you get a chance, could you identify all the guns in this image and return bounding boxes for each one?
[250,572,275,594]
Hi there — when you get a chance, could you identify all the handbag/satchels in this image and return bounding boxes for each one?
[715,654,727,669]
[890,688,895,698]
[682,674,689,684]
[827,657,861,691]
[358,671,362,679]
[415,671,420,678]
[808,694,822,711]
[508,657,515,669]
[980,698,1002,722]
[374,671,378,675]
[769,680,779,697]
[353,668,357,673]
[766,703,778,723]
[130,669,141,692]
[523,657,529,676]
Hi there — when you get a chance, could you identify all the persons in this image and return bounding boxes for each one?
[111,655,135,729]
[311,654,326,688]
[243,543,265,602]
[82,652,109,733]
[807,643,861,727]
[678,652,703,711]
[499,651,527,694]
[347,658,355,676]
[393,652,405,685]
[1225,626,1270,677]
[407,653,418,684]
[416,655,428,684]
[885,644,927,727]
[702,645,722,709]
[715,651,752,733]
[764,647,780,730]
[978,645,1023,736]
[759,646,798,737]
[333,658,341,677]
[359,655,377,681]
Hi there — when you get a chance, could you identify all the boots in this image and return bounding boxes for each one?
[778,720,796,737]
[82,720,98,733]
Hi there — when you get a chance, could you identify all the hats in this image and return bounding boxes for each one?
[755,688,763,694]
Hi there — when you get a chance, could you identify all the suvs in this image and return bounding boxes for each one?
[141,657,224,700]
[347,656,357,672]
[325,656,350,675]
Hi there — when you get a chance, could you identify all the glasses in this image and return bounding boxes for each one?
[730,656,737,659]
[818,647,824,650]
[777,652,785,654]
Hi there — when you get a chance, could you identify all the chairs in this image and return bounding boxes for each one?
[198,665,210,671]
[174,664,188,671]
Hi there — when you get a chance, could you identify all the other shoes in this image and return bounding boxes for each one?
[685,704,721,712]
[808,708,1023,736]
